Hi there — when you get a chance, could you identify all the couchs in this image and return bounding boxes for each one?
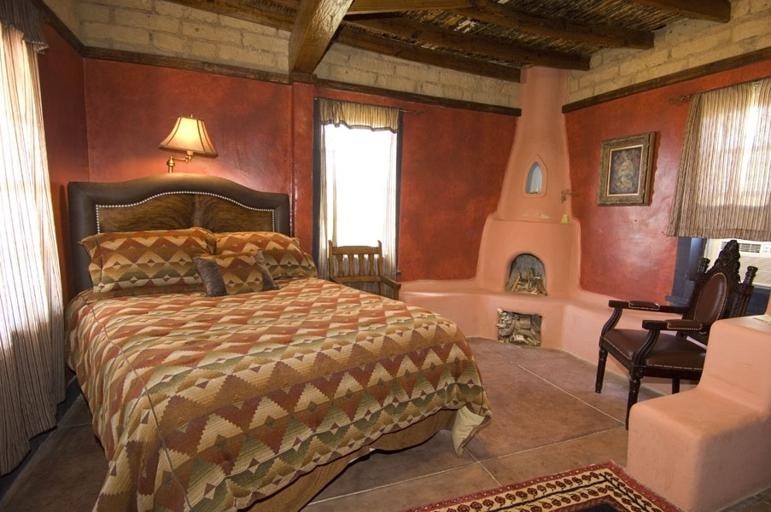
[628,295,771,511]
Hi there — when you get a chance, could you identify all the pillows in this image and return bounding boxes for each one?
[214,231,319,280]
[75,226,217,302]
[194,249,280,295]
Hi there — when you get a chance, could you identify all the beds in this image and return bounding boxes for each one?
[66,173,491,512]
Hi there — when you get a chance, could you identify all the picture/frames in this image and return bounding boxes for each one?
[595,131,656,206]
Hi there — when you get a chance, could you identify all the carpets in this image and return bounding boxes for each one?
[406,462,681,512]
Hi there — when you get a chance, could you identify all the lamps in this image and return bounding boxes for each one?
[158,113,218,172]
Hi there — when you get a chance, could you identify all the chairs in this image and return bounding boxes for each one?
[327,240,402,301]
[595,239,759,432]
